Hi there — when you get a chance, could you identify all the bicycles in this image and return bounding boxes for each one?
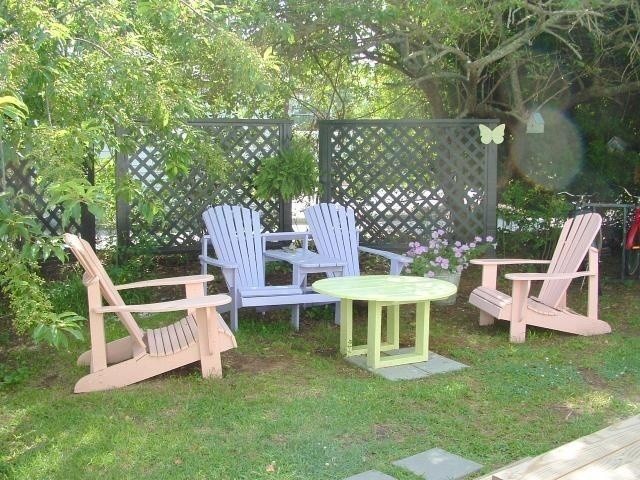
[554,186,640,291]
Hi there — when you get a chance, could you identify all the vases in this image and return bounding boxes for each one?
[424,265,464,307]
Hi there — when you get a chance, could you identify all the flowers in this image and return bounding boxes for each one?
[395,224,494,275]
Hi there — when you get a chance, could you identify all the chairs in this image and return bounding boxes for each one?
[62,229,239,395]
[287,203,414,337]
[470,213,611,343]
[198,200,317,334]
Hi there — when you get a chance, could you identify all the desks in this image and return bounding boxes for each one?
[310,275,458,368]
